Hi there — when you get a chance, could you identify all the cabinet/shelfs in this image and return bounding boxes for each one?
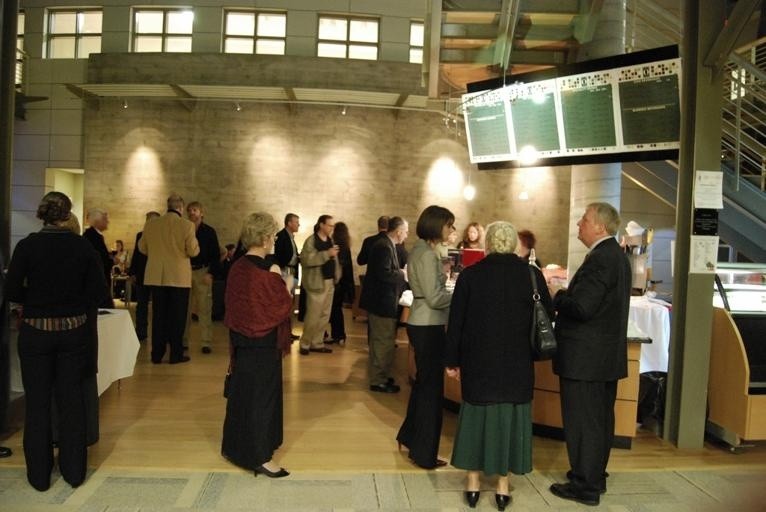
[702,262,766,454]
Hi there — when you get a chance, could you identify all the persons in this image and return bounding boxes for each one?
[129,193,247,364]
[219,211,296,479]
[445,221,555,511]
[547,202,632,505]
[395,204,454,470]
[267,215,354,355]
[357,216,407,348]
[62,207,130,311]
[5,191,94,490]
[1,446,13,459]
[52,211,99,449]
[358,215,409,394]
[456,222,541,270]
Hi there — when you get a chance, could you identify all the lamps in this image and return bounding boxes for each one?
[341,106,348,115]
[121,98,128,109]
[233,102,241,112]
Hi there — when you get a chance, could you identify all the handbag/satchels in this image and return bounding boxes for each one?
[531,300,558,361]
[224,374,232,398]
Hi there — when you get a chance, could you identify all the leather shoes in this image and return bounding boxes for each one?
[566,470,607,495]
[291,335,300,339]
[370,385,400,393]
[387,377,393,383]
[180,356,190,361]
[299,348,310,355]
[1,447,11,456]
[550,484,599,506]
[310,348,332,352]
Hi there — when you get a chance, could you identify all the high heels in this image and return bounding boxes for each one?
[466,491,479,507]
[396,440,410,452]
[409,455,446,469]
[324,336,339,344]
[254,459,289,477]
[340,335,347,341]
[495,495,512,512]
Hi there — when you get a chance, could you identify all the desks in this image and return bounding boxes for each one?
[9,303,141,400]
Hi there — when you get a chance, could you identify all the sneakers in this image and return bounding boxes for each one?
[202,347,211,354]
